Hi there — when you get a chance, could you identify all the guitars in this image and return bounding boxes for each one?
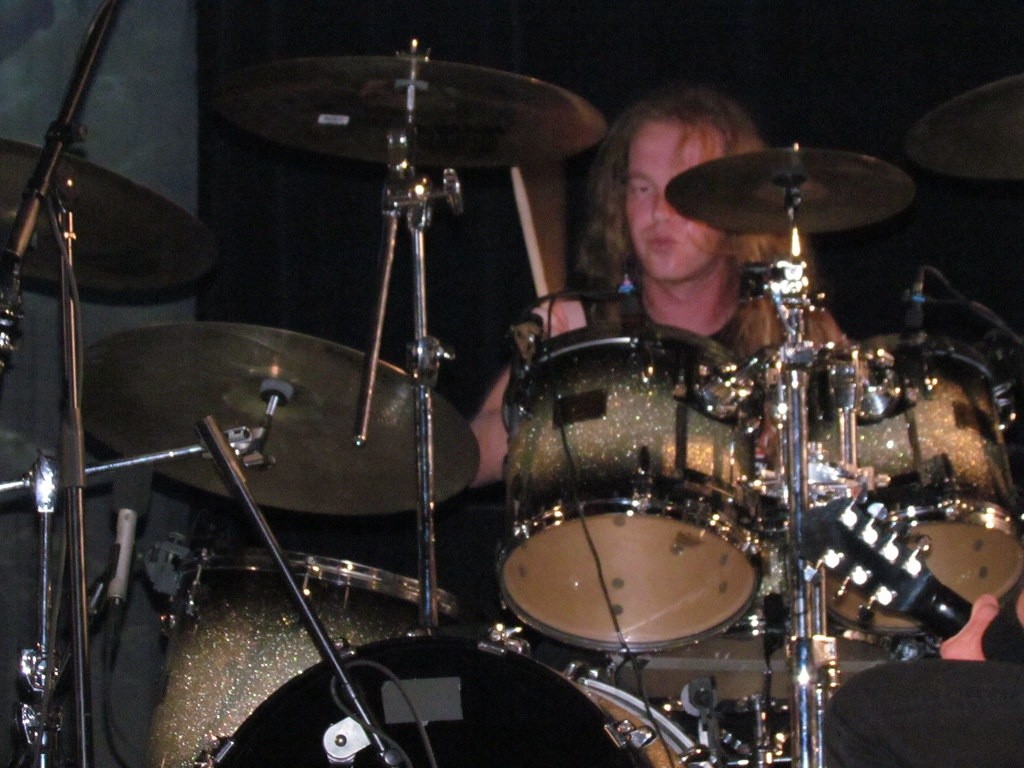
[784,496,1024,663]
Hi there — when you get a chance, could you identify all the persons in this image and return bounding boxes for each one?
[466,85,847,488]
[821,590,1024,768]
[0,0,198,768]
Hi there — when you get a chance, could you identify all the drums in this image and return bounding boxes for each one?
[566,471,893,703]
[777,332,1024,632]
[206,632,722,768]
[150,547,457,768]
[501,318,763,654]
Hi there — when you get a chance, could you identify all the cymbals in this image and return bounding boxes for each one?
[900,75,1024,184]
[664,142,917,236]
[83,320,479,519]
[211,52,608,171]
[0,139,218,293]
